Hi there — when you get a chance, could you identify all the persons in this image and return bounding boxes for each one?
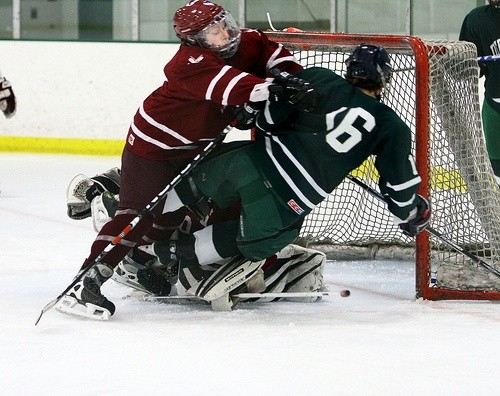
[56,0,430,321]
[453,0,500,176]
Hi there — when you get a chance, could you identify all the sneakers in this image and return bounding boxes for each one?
[66,168,123,220]
[55,261,117,319]
[109,248,171,297]
[91,190,122,231]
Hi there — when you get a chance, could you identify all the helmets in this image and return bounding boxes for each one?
[343,42,393,87]
[173,0,241,58]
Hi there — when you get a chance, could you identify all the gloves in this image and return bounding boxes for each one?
[268,71,316,112]
[0,77,15,116]
[231,101,261,130]
[399,194,431,236]
[443,60,476,82]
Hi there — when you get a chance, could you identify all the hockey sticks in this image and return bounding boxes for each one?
[141,290,352,300]
[393,55,500,71]
[35,111,244,325]
[346,171,500,277]
